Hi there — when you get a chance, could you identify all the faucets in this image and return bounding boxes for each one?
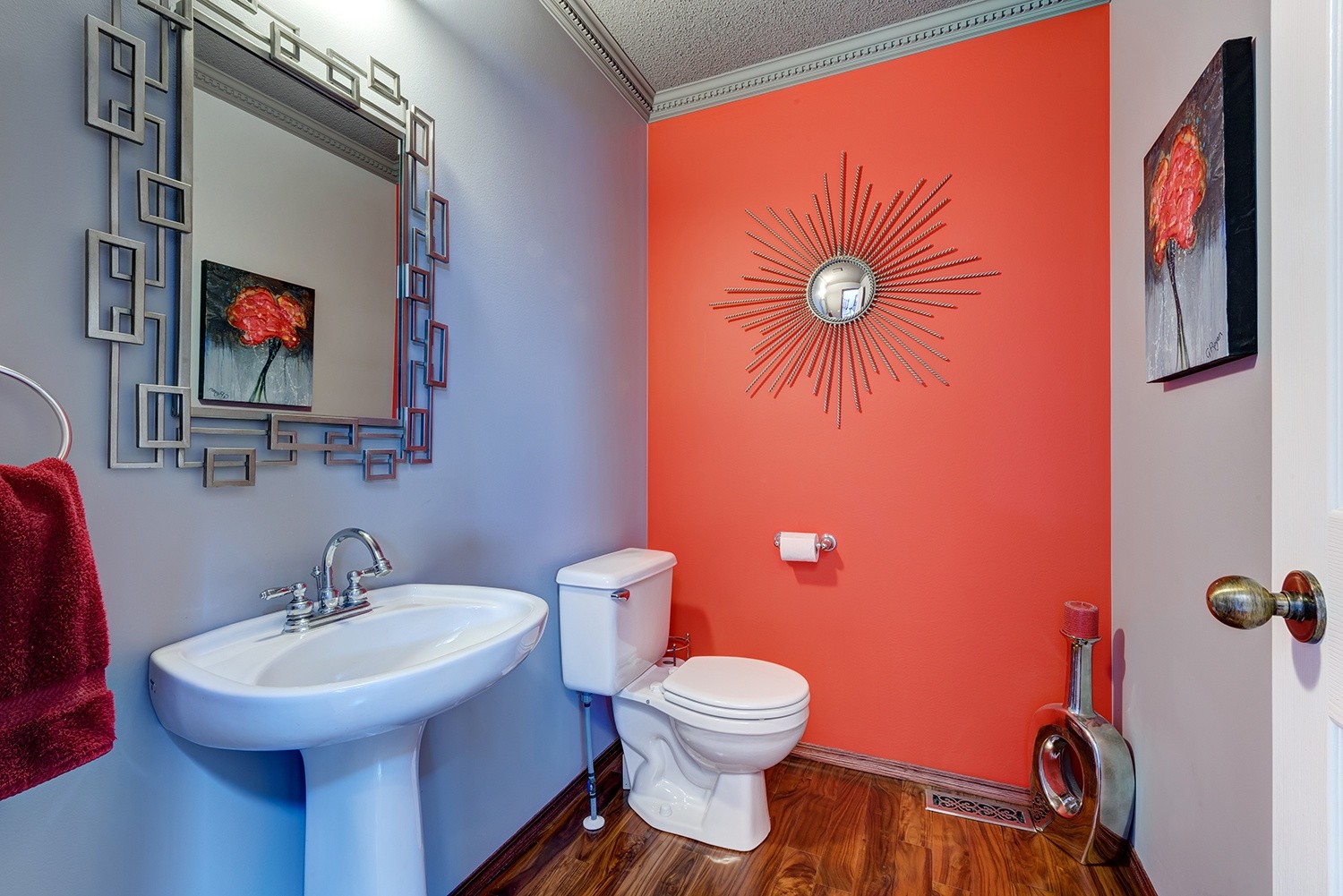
[319,526,392,614]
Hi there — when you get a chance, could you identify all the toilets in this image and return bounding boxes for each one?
[556,548,812,852]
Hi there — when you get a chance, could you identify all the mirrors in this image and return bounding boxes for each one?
[75,0,452,488]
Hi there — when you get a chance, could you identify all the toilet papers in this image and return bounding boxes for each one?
[779,531,820,562]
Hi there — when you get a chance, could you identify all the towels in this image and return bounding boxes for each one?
[0,455,116,800]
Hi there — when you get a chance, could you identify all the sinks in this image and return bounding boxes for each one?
[149,580,550,751]
